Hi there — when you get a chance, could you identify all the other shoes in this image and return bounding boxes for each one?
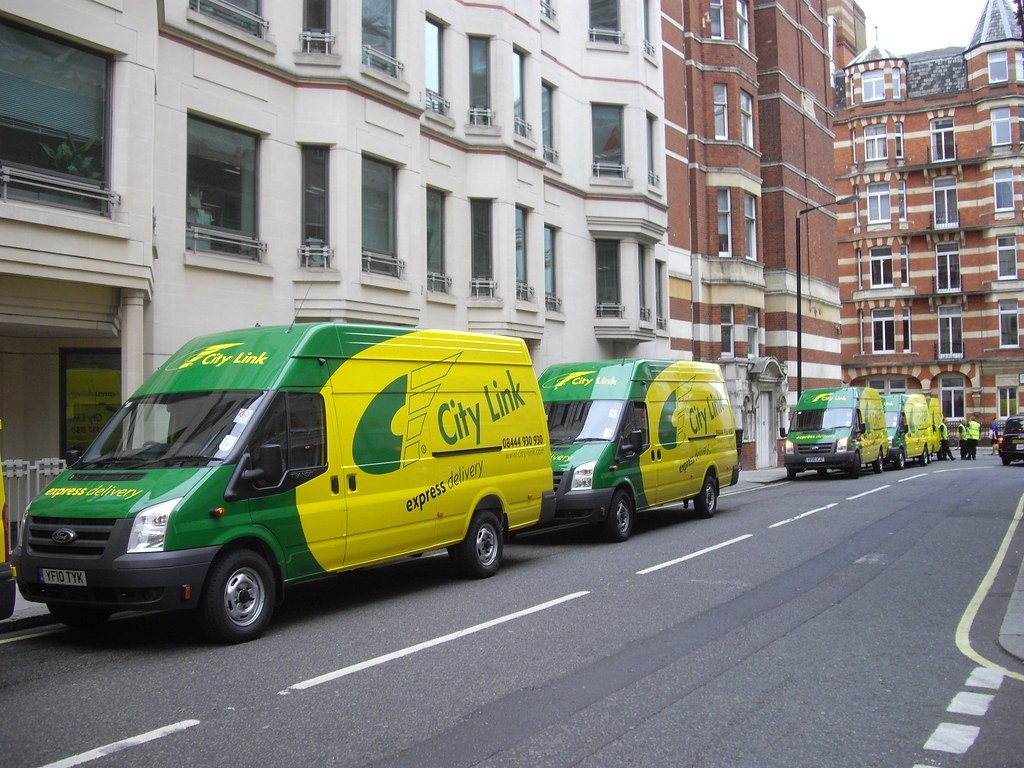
[938,453,978,461]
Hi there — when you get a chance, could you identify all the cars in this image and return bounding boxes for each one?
[997,414,1024,466]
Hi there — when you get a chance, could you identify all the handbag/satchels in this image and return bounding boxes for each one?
[994,440,999,449]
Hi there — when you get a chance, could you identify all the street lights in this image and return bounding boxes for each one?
[794,192,860,406]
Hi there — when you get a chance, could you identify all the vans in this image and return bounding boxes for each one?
[536,356,740,539]
[19,322,557,642]
[780,385,888,479]
[876,392,943,467]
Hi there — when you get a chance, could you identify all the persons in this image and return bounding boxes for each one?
[967,416,981,460]
[960,418,968,459]
[991,416,1000,455]
[940,418,955,460]
